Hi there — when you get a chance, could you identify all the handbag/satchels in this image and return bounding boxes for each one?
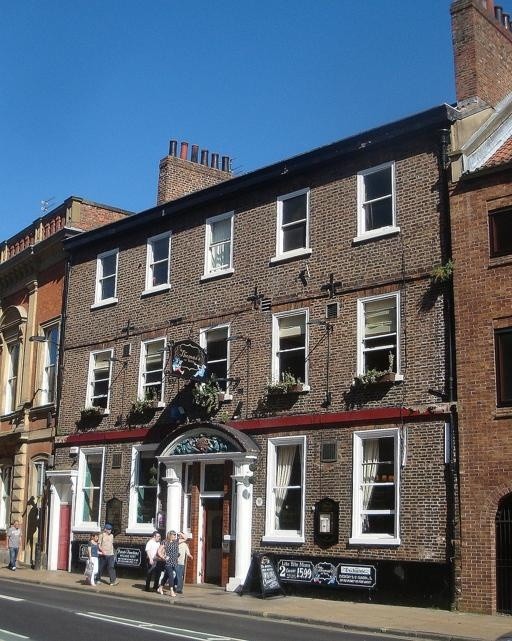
[153,554,169,561]
[84,548,89,557]
[84,563,94,577]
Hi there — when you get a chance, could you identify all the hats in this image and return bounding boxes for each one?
[104,524,113,529]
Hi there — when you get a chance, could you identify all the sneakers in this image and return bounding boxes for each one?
[7,565,16,571]
[84,580,184,597]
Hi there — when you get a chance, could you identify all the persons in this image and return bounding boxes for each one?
[6,520,22,571]
[144,530,193,597]
[94,524,120,586]
[87,532,105,586]
